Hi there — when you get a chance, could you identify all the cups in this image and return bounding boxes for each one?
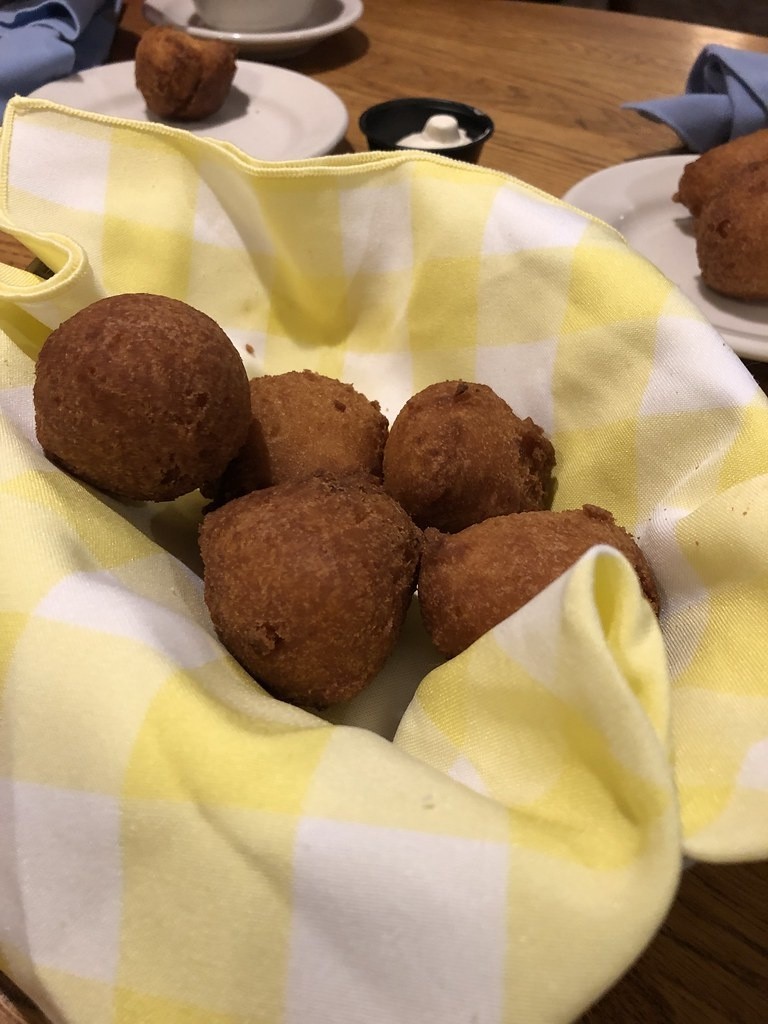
[358,95,494,169]
[193,0,316,32]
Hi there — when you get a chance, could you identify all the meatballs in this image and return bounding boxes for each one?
[134,24,235,122]
[672,128,768,305]
[33,292,660,706]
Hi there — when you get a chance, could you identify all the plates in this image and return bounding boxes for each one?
[141,0,362,57]
[28,60,349,162]
[558,155,768,359]
